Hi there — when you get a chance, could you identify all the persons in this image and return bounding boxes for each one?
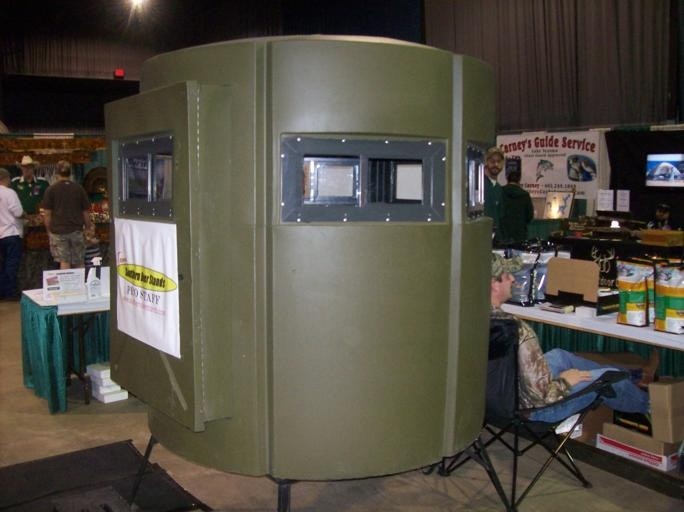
[41,160,91,270]
[6,154,51,229]
[489,251,660,424]
[482,147,504,249]
[0,167,35,302]
[500,169,533,246]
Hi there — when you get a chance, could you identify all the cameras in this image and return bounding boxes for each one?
[644,152,684,188]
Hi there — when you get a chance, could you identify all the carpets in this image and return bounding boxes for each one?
[0,439,216,512]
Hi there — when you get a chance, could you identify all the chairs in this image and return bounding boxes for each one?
[438,318,629,512]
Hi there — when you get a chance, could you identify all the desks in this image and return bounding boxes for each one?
[22,288,113,406]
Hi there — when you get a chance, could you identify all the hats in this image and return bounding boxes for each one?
[491,253,522,276]
[486,147,503,159]
[16,155,39,167]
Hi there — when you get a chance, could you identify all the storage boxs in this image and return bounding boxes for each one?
[597,376,684,473]
